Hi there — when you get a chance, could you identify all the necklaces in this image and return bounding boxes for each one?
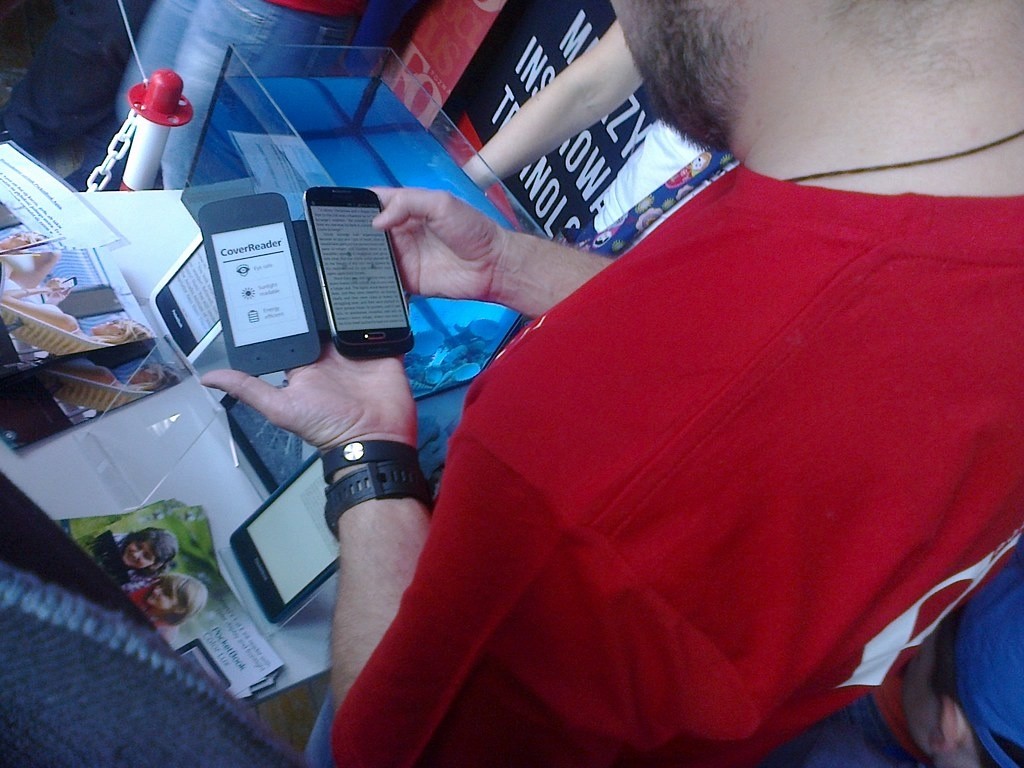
[783,129,1024,182]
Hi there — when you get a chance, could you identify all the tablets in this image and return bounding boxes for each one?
[148,232,223,366]
[229,450,342,624]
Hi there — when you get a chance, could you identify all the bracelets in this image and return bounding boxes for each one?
[321,440,419,485]
[325,461,433,542]
[24,289,29,298]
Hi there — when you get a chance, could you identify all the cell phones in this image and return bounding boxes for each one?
[302,187,416,362]
[60,277,77,288]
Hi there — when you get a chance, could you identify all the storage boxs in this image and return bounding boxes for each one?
[180,43,550,414]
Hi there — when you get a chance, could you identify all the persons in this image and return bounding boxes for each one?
[34,358,180,411]
[758,531,1024,768]
[0,232,48,252]
[121,574,208,645]
[200,0,1024,768]
[76,526,178,587]
[0,253,151,355]
[0,0,368,191]
[462,17,710,245]
